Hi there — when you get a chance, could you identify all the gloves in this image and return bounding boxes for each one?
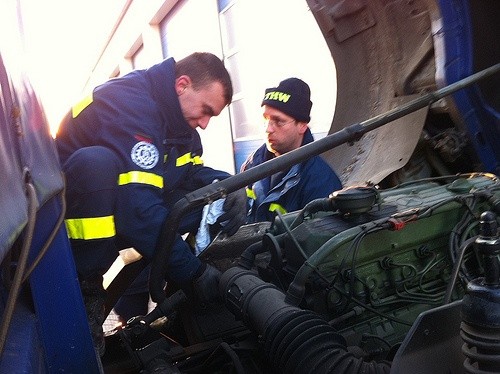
[217,187,248,236]
[193,264,222,302]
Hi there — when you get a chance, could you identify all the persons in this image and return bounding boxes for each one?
[96,76,343,325]
[51,50,251,311]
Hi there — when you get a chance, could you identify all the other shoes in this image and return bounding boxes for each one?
[80,288,107,358]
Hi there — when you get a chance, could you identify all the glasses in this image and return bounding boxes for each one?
[264,118,294,127]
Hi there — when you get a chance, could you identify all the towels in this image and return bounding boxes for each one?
[194,178,226,257]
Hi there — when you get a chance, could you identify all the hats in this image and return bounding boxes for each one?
[261,78,312,123]
